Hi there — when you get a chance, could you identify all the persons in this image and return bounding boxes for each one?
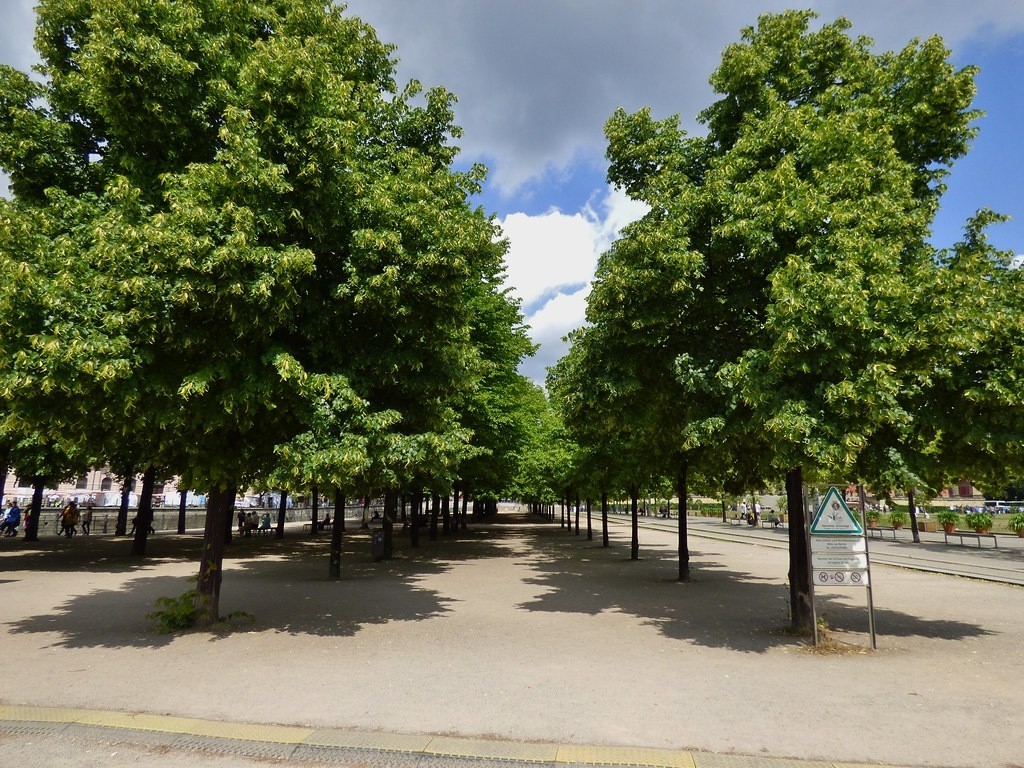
[368,511,379,524]
[915,504,1024,515]
[626,500,783,529]
[0,501,330,539]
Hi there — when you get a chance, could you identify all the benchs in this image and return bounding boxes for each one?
[942,531,1000,552]
[730,518,775,530]
[238,526,281,536]
[655,511,669,518]
[636,510,646,515]
[301,522,335,532]
[866,527,896,541]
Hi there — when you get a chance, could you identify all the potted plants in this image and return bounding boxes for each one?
[936,510,961,534]
[965,511,994,535]
[887,509,908,530]
[700,506,725,518]
[862,508,882,528]
[1004,508,1024,540]
